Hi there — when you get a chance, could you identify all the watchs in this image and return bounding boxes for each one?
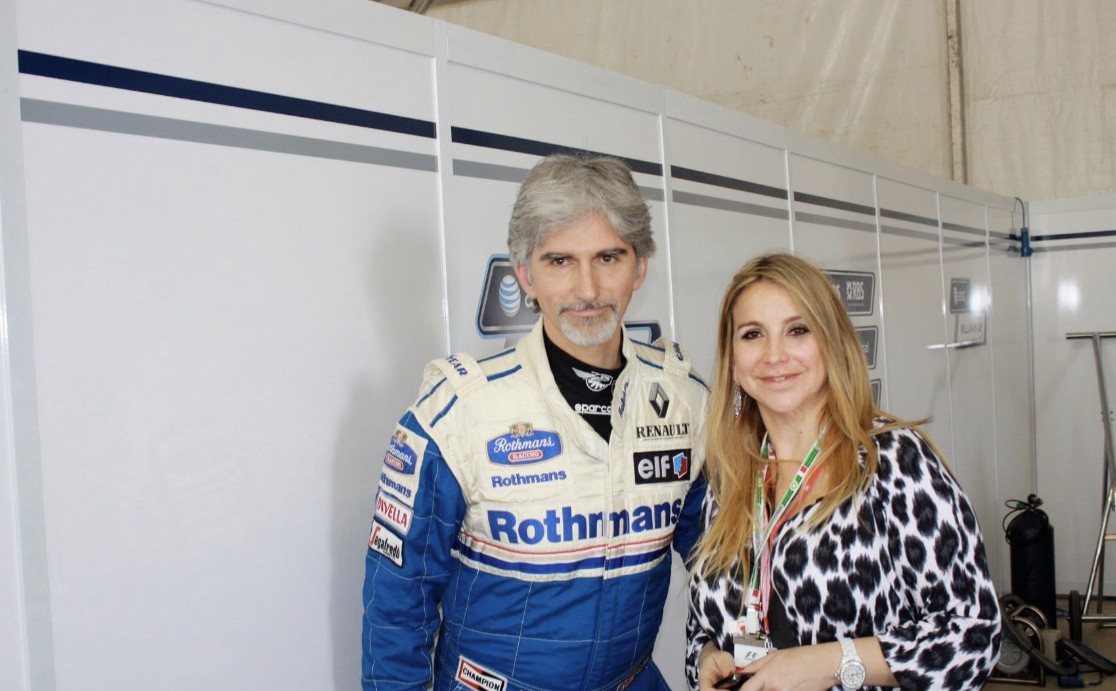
[834,637,866,691]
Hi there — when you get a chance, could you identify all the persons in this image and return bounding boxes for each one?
[361,153,719,691]
[685,253,1002,691]
